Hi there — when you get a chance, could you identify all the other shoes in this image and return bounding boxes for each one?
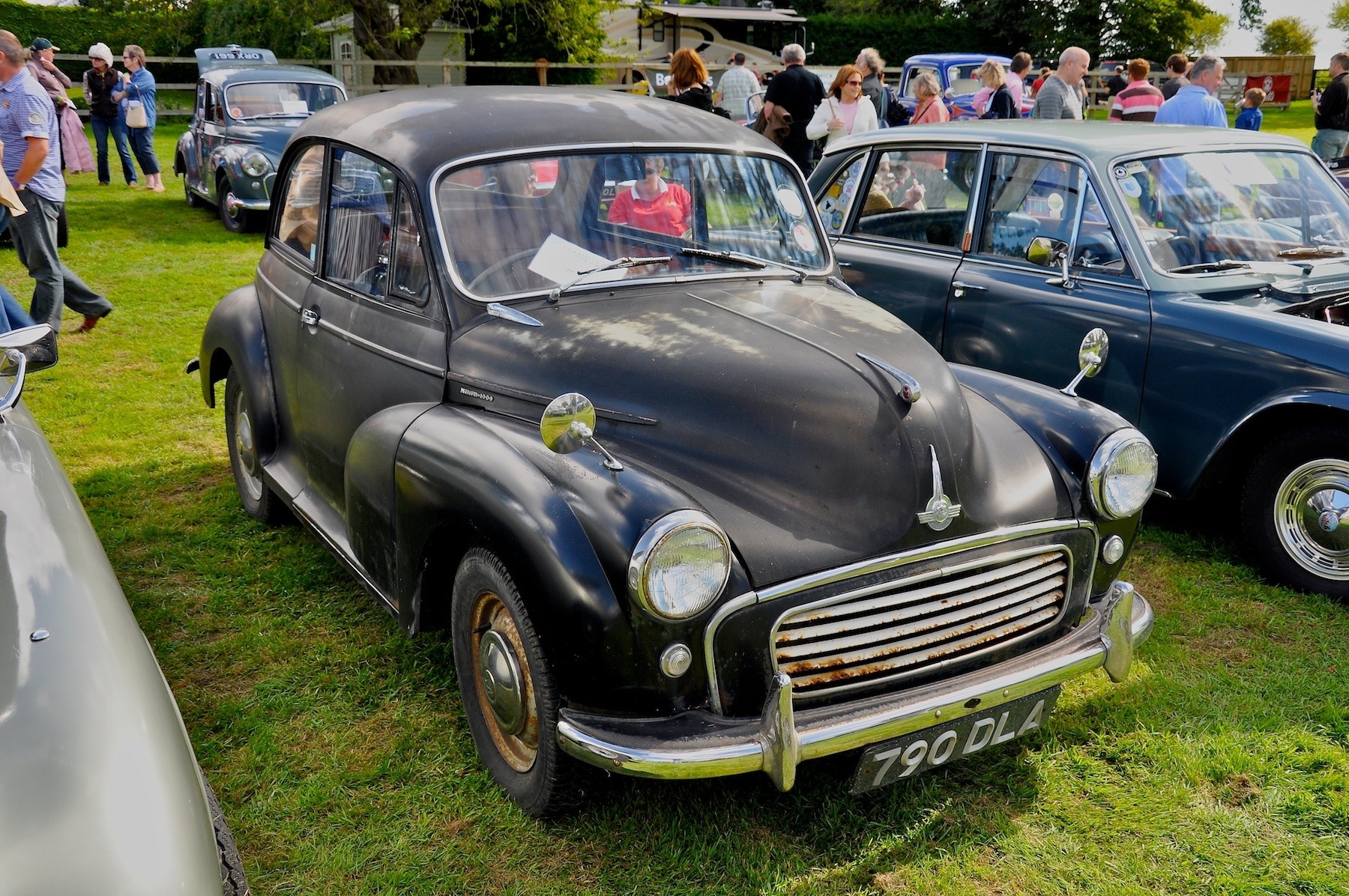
[99,182,107,187]
[129,182,137,188]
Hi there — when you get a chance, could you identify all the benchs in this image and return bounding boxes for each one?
[334,201,585,280]
[210,100,283,120]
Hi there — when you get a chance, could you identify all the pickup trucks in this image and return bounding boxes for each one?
[884,53,1038,126]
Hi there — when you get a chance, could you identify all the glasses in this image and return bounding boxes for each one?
[646,167,659,175]
[1165,65,1170,69]
[89,56,104,61]
[845,79,863,87]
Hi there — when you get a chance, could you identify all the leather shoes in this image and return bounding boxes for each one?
[69,308,113,334]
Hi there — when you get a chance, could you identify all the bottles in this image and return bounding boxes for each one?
[1235,99,1245,109]
[1316,92,1322,106]
[371,257,388,296]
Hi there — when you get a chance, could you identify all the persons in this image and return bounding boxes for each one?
[1311,52,1349,160]
[1106,65,1127,119]
[609,155,713,236]
[111,44,166,193]
[0,29,113,335]
[83,42,138,188]
[716,53,762,122]
[977,58,1014,196]
[763,43,828,180]
[972,51,1032,117]
[1109,58,1165,226]
[1031,46,1091,213]
[1153,54,1228,230]
[806,64,891,174]
[1235,88,1266,131]
[26,37,72,187]
[1161,54,1190,100]
[855,47,888,121]
[656,47,713,112]
[908,73,950,209]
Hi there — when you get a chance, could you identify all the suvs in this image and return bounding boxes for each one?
[183,83,1158,823]
[1087,59,1170,104]
[173,44,381,234]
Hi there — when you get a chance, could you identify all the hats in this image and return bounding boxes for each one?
[88,42,114,69]
[30,36,61,51]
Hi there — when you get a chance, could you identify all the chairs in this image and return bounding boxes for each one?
[1132,213,1183,271]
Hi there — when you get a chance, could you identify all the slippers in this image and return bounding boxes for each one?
[153,189,165,193]
[143,185,155,190]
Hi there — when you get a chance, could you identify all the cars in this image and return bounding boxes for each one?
[677,117,1349,599]
[0,324,250,896]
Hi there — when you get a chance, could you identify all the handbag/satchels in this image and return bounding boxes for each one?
[888,85,910,125]
[126,85,148,128]
[815,98,836,150]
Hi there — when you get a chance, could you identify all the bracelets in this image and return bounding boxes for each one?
[11,179,26,189]
[58,96,62,104]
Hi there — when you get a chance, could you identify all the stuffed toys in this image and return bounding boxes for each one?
[898,179,926,211]
